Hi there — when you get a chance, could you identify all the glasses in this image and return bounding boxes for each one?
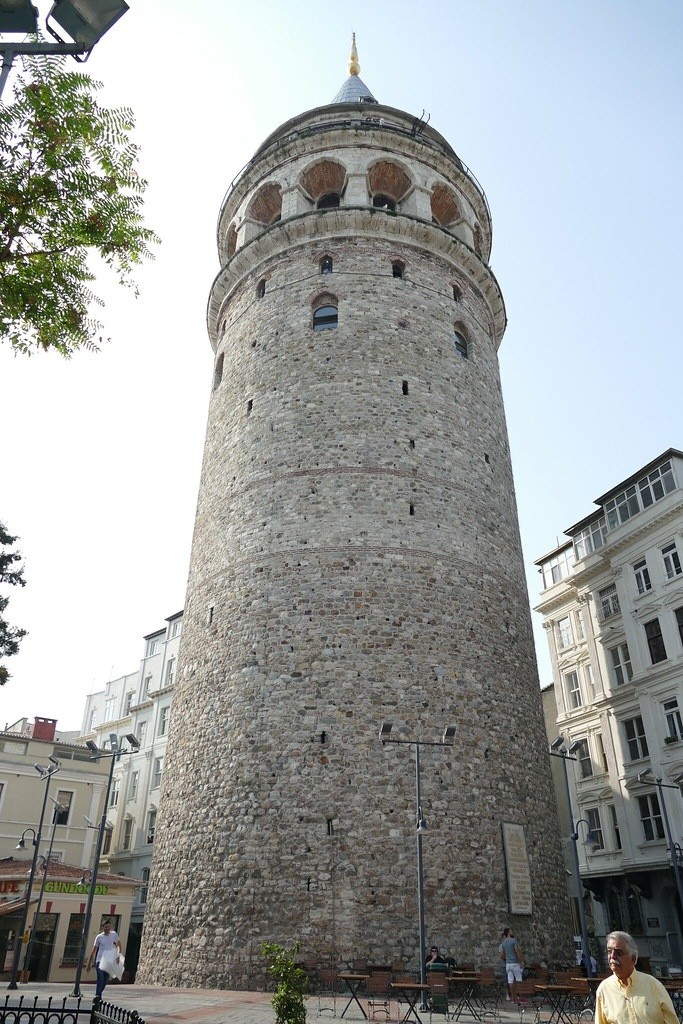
[605,948,631,957]
[431,950,437,952]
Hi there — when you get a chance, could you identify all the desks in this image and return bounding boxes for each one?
[523,967,545,978]
[391,981,430,1023]
[444,977,481,1021]
[452,971,487,1010]
[572,978,606,1008]
[535,985,579,1024]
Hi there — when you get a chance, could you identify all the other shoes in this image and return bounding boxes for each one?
[519,998,528,1004]
[506,994,511,1000]
[512,998,516,1003]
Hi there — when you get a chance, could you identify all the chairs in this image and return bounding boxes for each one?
[481,966,502,1023]
[514,980,540,1024]
[317,969,337,1018]
[365,977,389,1024]
[396,974,418,1024]
[567,967,592,1008]
[535,969,550,1003]
[552,971,571,1007]
[426,973,447,1024]
[371,971,391,1020]
[338,974,369,1020]
[529,977,557,1024]
[464,962,475,972]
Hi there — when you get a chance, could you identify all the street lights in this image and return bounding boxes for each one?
[548,734,598,1006]
[380,719,462,1015]
[7,752,62,992]
[636,766,683,912]
[67,733,143,999]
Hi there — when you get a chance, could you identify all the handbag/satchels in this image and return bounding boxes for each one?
[98,949,125,981]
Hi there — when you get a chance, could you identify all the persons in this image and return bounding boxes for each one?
[580,950,597,979]
[499,928,528,1004]
[86,920,122,1005]
[423,946,449,978]
[595,931,679,1024]
